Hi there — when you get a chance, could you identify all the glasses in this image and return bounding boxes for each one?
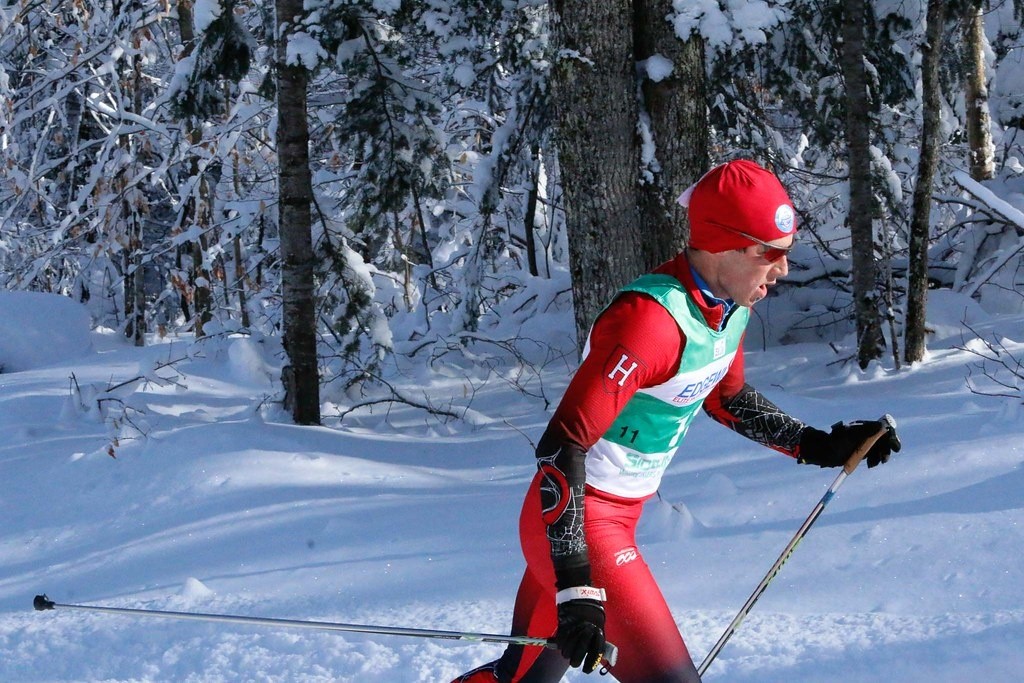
[707,221,795,264]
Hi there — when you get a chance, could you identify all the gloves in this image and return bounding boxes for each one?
[555,574,607,674]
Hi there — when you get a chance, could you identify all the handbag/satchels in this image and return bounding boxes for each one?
[797,419,900,469]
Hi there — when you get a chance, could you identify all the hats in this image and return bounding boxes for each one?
[674,160,797,254]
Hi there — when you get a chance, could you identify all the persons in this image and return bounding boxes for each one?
[446,160,902,683]
